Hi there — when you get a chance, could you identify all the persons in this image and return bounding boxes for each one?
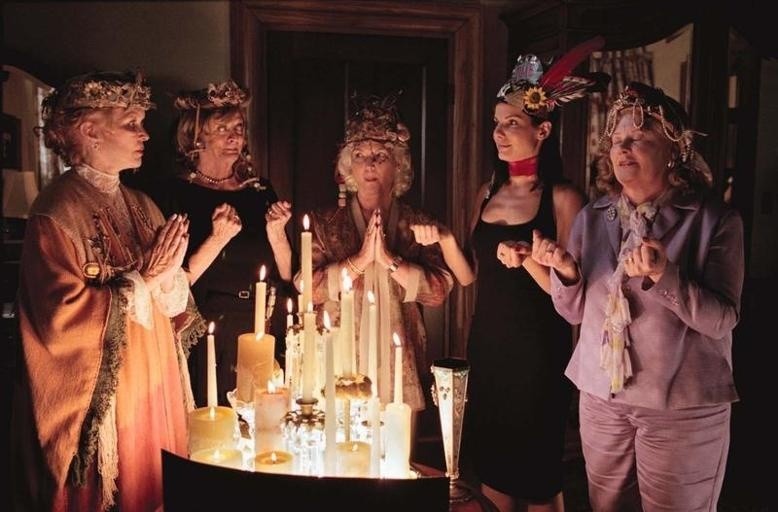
[408,35,603,512]
[293,88,455,460]
[531,81,746,512]
[153,78,299,408]
[7,64,208,512]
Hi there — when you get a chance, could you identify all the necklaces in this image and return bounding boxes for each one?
[193,164,237,185]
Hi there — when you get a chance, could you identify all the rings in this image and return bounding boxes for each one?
[628,257,634,263]
[382,234,388,240]
[235,215,240,221]
[367,233,371,237]
[269,210,273,216]
[546,249,554,255]
[500,253,505,258]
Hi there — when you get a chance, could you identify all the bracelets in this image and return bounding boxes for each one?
[387,255,403,274]
[347,256,367,276]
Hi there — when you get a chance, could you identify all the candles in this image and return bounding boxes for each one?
[301,214,313,315]
[206,320,218,408]
[392,332,404,404]
[366,289,378,397]
[254,379,291,450]
[302,300,317,400]
[370,381,381,478]
[334,440,371,476]
[384,395,412,478]
[254,264,267,337]
[188,404,241,448]
[235,329,275,403]
[339,268,357,378]
[189,447,243,471]
[285,298,294,387]
[254,449,294,475]
[323,310,337,477]
